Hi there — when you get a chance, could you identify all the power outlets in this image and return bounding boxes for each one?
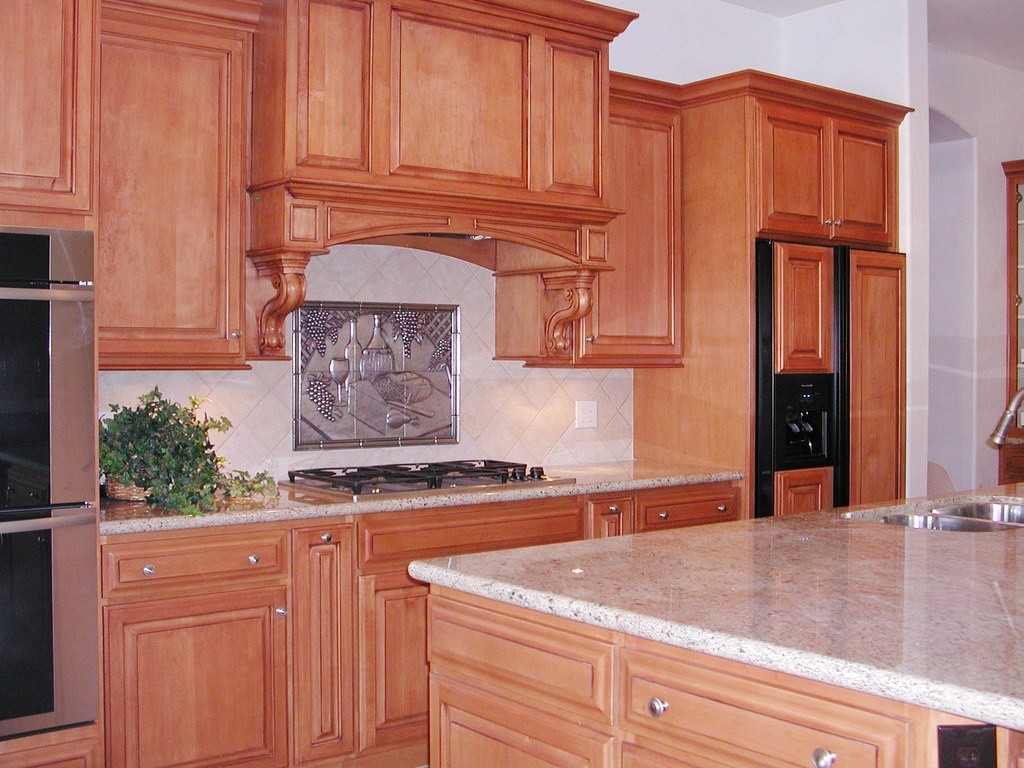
[576,400,598,429]
[937,724,997,768]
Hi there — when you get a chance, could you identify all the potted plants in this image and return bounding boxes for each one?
[98,382,284,518]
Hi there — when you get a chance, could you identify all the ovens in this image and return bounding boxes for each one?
[0,225,101,740]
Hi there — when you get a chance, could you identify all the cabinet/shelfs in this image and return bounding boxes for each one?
[0,0,1024,768]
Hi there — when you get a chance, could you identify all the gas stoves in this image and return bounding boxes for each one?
[279,460,577,505]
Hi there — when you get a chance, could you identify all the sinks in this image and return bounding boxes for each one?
[929,500,1024,524]
[878,512,1024,541]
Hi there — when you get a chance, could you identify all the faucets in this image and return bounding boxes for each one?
[989,386,1024,445]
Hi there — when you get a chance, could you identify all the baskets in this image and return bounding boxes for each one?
[105,463,155,501]
